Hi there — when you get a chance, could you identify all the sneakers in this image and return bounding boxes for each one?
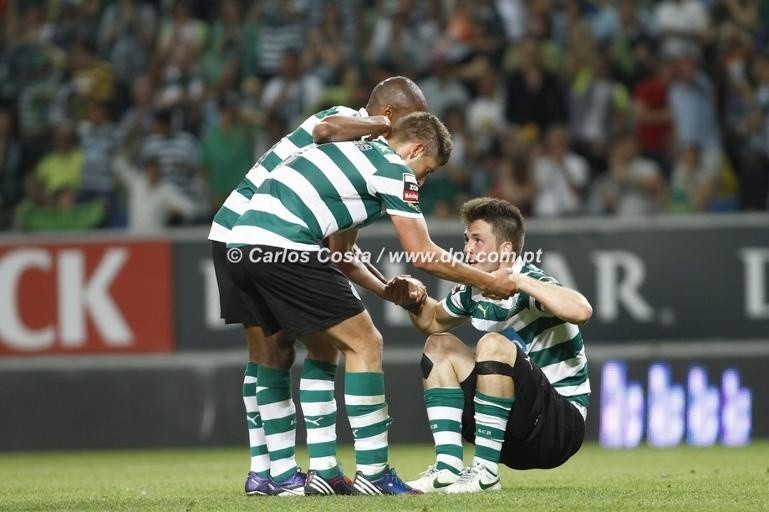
[246,469,308,497]
[348,465,425,497]
[403,464,503,494]
[304,470,353,497]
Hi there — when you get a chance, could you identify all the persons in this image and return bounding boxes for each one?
[207,74,426,492]
[387,194,595,495]
[222,111,517,495]
[1,1,768,230]
